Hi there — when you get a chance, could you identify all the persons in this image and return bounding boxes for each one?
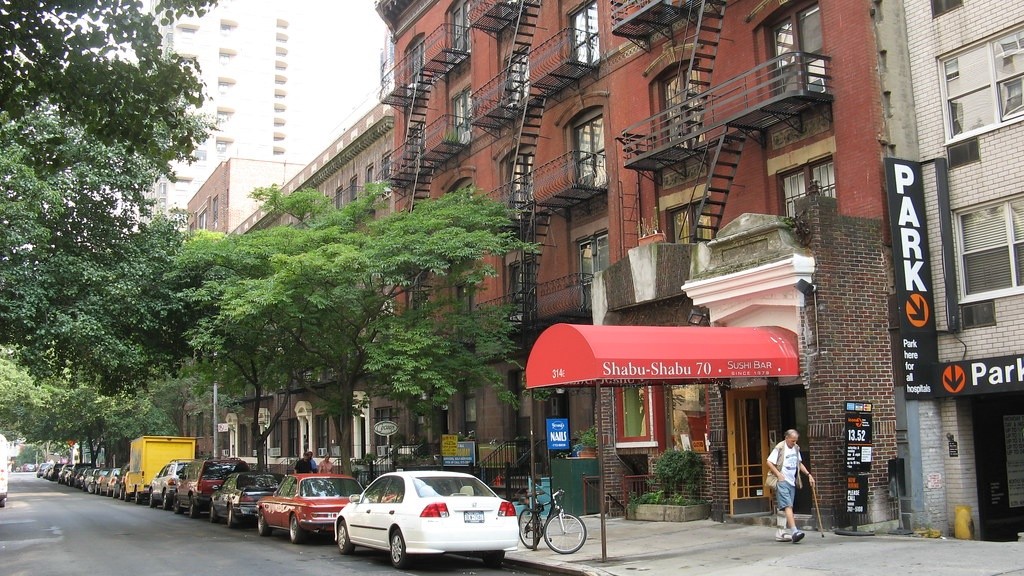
[766,429,815,542]
[318,454,337,475]
[308,451,317,473]
[294,452,313,474]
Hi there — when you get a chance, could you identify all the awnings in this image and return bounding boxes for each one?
[525,322,798,562]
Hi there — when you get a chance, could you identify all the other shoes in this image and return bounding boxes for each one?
[792,531,805,542]
[776,533,792,542]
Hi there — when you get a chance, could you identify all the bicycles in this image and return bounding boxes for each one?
[518,487,587,555]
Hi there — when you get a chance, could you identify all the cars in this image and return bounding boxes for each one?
[18,462,130,500]
[334,470,520,570]
[209,470,282,529]
[255,473,365,545]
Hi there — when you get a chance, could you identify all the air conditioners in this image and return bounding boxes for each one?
[407,83,420,99]
[377,445,390,456]
[253,450,258,457]
[268,447,281,457]
[221,449,230,457]
[318,448,327,456]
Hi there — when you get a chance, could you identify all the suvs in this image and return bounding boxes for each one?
[148,458,197,510]
[173,457,251,519]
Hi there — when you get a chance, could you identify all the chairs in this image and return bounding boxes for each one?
[460,486,474,496]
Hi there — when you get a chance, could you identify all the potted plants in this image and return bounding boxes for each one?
[574,423,598,459]
[637,216,667,246]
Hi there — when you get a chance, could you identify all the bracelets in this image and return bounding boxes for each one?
[807,472,810,476]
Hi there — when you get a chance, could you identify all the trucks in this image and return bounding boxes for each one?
[124,435,196,505]
[0,434,12,508]
